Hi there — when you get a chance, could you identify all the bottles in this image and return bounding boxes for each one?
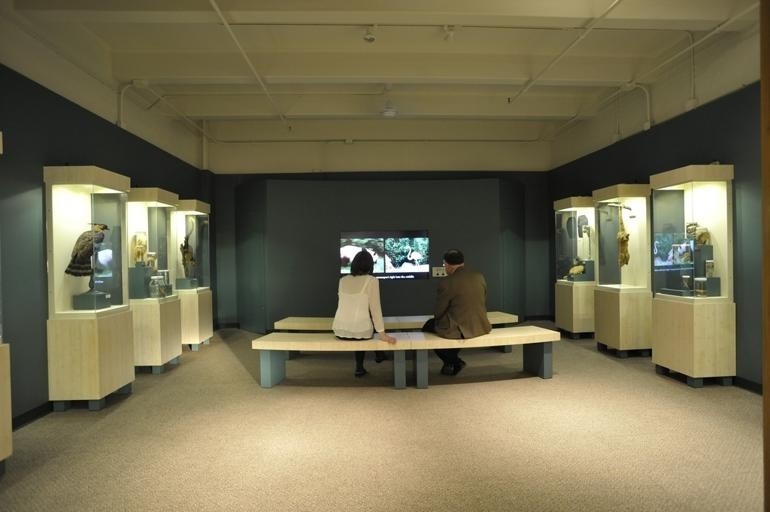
[149,276,165,297]
[157,270,169,285]
[147,252,157,273]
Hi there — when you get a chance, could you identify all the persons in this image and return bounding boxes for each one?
[422,249,491,376]
[332,251,397,377]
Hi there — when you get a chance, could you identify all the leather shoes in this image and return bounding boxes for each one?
[440,364,451,375]
[452,358,466,375]
[376,355,386,363]
[356,369,366,376]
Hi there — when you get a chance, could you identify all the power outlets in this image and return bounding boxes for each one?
[432,267,448,277]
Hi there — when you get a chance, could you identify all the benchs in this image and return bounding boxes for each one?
[274,317,399,360]
[411,326,561,389]
[252,333,411,388]
[398,311,518,329]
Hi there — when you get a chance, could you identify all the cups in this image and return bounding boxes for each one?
[682,275,690,289]
[705,259,714,277]
[695,278,707,297]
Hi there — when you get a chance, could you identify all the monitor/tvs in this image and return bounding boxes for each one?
[336,229,432,280]
